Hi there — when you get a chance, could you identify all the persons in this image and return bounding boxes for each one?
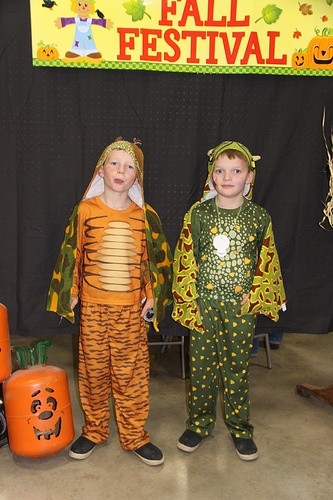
[46,141,173,466]
[171,141,287,460]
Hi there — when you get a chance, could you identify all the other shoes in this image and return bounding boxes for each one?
[264,335,280,349]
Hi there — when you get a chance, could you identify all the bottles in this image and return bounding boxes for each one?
[144,310,154,322]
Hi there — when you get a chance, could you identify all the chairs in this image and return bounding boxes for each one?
[253,325,272,369]
[148,303,187,379]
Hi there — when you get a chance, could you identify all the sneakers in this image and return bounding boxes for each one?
[176,427,207,453]
[230,428,260,462]
[68,433,110,459]
[131,442,167,465]
